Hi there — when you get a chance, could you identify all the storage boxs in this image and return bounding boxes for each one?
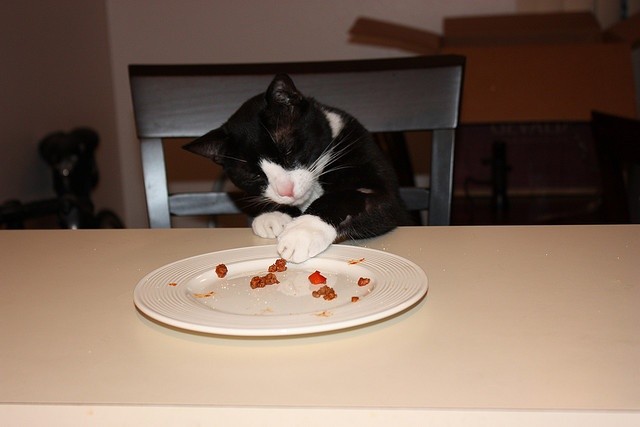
[348,11,639,190]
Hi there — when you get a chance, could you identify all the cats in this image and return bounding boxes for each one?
[181,70,414,264]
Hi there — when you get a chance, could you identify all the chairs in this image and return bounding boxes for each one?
[127,54,465,224]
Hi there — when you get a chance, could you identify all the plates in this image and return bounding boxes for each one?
[133,242,429,337]
